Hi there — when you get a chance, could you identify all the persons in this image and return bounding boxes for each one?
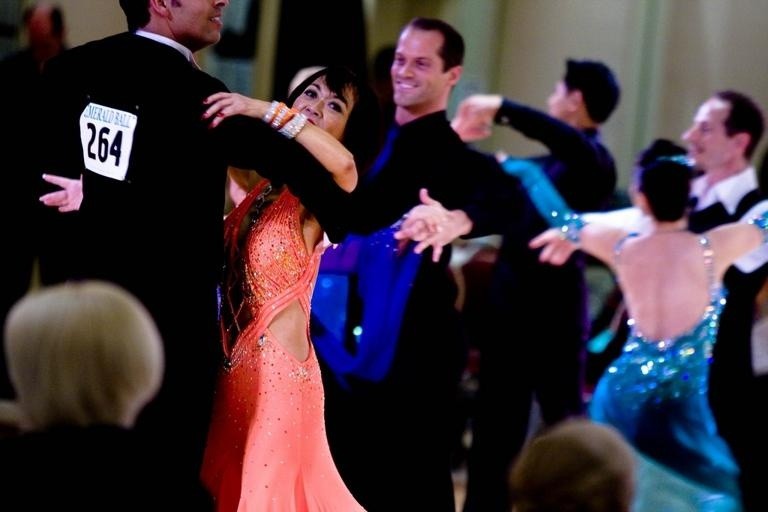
[1,0,767,512]
